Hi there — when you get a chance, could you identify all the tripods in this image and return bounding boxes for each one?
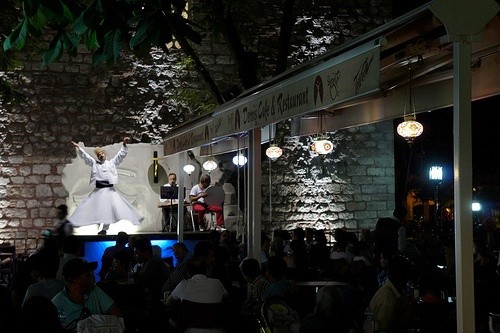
[160,186,186,232]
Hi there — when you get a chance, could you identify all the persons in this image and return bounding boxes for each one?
[47,203,72,235]
[394,176,437,258]
[0,231,272,333]
[160,173,188,231]
[266,205,500,333]
[67,138,144,234]
[189,175,227,231]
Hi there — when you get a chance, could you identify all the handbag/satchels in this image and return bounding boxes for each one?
[77,313,126,333]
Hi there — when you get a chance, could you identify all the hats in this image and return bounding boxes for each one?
[62,258,98,281]
[116,232,129,243]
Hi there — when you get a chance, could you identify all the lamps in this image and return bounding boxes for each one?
[311,109,334,155]
[232,138,248,168]
[221,171,236,196]
[396,61,424,144]
[266,123,283,161]
[202,143,217,174]
[183,149,195,176]
